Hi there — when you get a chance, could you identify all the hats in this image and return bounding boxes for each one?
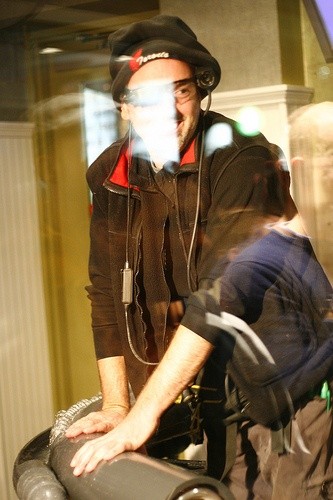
[106,12,221,103]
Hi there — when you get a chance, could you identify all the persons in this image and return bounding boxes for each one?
[220,100,333,499]
[67,13,291,474]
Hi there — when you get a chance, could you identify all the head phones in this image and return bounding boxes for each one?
[194,64,217,90]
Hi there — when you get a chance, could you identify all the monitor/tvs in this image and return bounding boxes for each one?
[304,0,333,64]
